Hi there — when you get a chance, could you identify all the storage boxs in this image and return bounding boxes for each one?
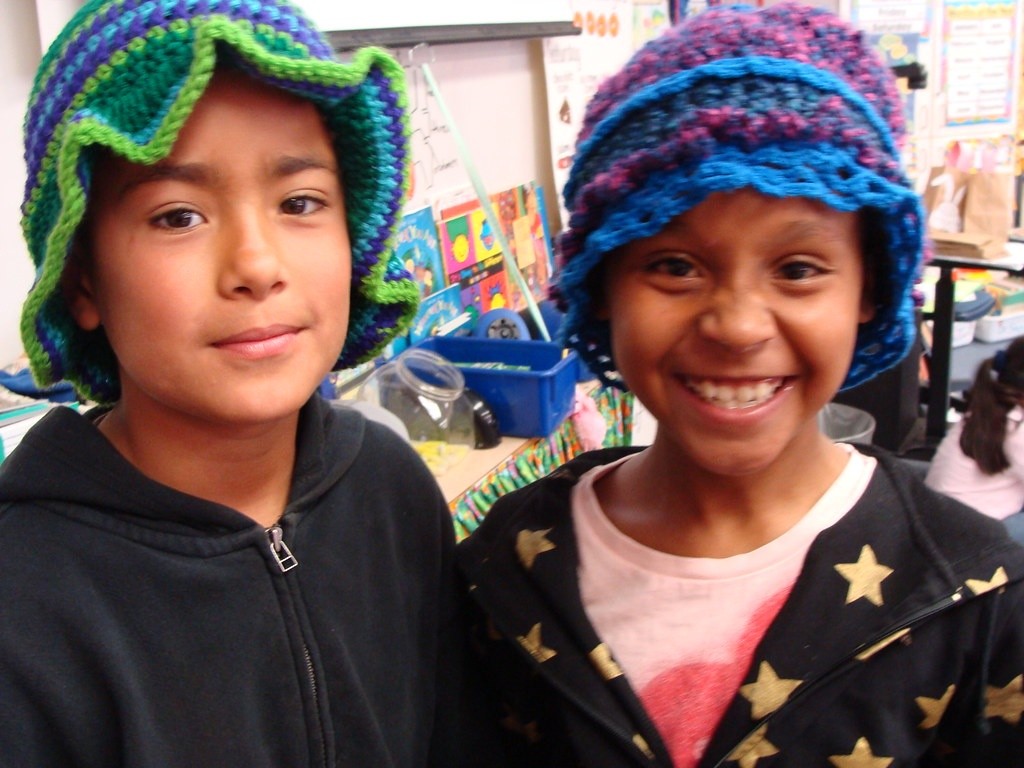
[410,337,578,439]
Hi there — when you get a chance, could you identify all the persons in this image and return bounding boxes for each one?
[921,336,1024,546]
[427,0,1024,768]
[0,0,455,768]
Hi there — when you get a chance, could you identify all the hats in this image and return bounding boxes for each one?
[546,4,918,396]
[18,0,421,406]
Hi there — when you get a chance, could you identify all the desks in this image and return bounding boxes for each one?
[894,238,1024,462]
